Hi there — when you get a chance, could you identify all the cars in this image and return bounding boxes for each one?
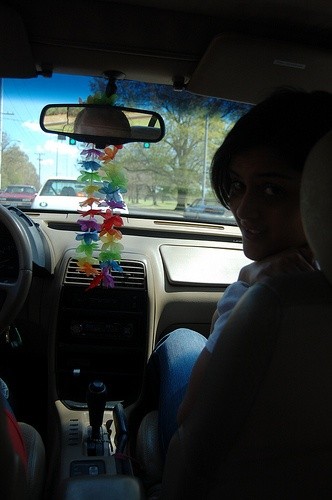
[185,198,240,229]
[29,177,130,216]
[0,184,38,202]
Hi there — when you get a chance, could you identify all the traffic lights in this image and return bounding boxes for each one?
[69,137,76,144]
[144,142,151,148]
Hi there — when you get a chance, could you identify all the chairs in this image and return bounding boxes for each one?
[59,186,76,196]
[0,131,332,500]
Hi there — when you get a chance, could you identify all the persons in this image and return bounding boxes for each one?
[137,83,332,465]
